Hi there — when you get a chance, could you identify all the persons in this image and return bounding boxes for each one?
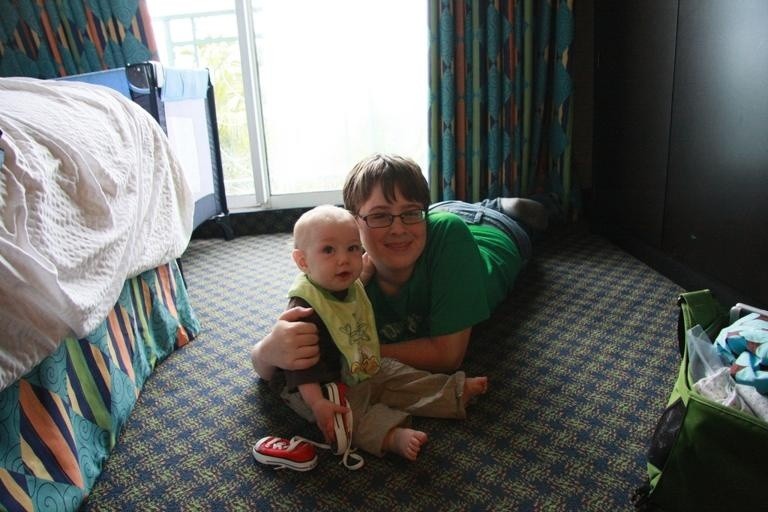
[276,203,489,461]
[249,151,562,384]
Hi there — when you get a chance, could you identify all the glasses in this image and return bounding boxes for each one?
[357,207,428,229]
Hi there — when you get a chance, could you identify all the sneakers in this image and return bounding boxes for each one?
[252,436,319,472]
[323,382,354,455]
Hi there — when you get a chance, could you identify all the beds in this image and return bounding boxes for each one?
[54,60,233,294]
[0,64,205,512]
